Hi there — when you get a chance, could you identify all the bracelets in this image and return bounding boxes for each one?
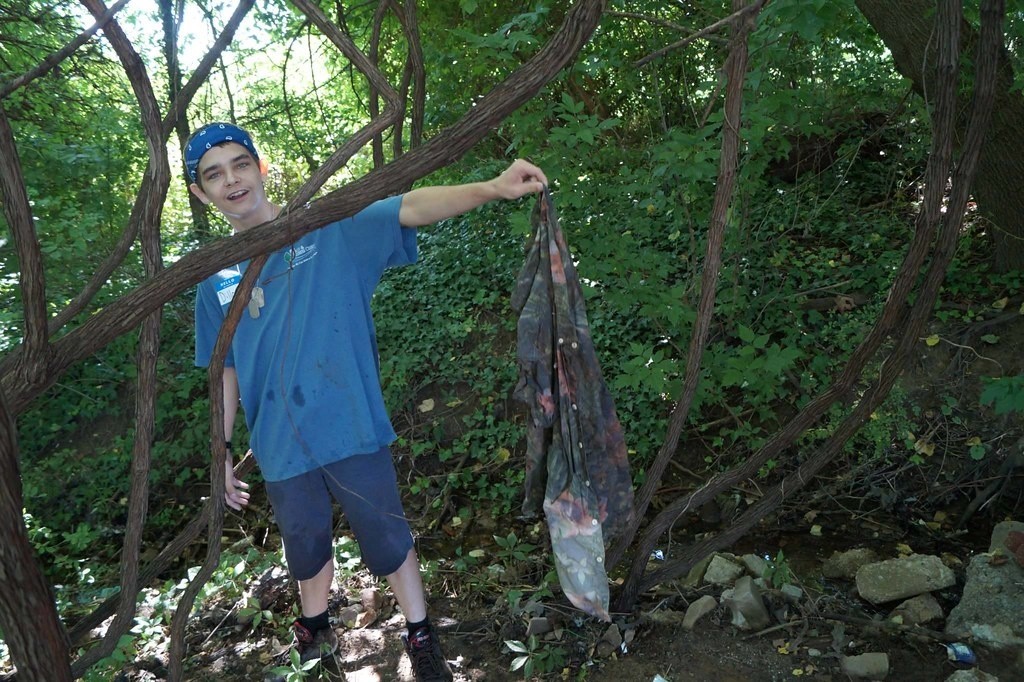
[207,438,233,451]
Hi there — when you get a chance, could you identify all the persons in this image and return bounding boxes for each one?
[180,121,548,682]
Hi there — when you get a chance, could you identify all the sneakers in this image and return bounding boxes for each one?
[272,618,338,670]
[400,623,454,682]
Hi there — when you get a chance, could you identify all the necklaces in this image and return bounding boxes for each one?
[231,201,276,319]
